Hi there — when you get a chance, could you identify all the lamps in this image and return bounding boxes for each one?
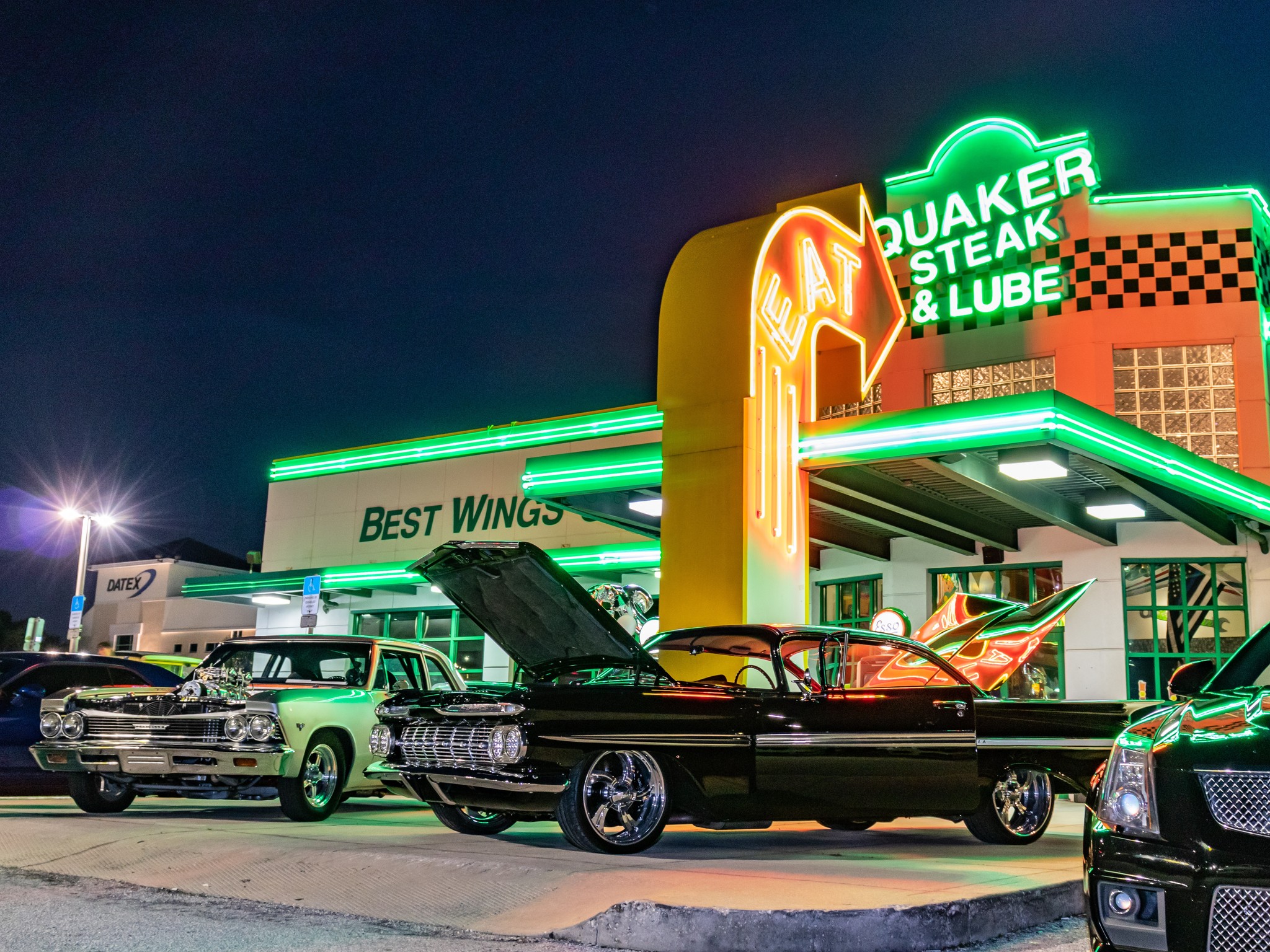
[1084,487,1146,522]
[627,495,663,518]
[996,443,1070,481]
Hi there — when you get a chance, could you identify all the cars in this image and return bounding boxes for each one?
[363,539,1162,856]
[0,651,186,795]
[1083,621,1270,952]
[27,637,470,821]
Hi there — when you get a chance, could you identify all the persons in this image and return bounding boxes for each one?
[97,641,114,657]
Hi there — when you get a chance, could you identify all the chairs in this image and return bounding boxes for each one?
[286,668,318,682]
[374,669,399,694]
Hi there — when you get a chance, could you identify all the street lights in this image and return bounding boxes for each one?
[60,505,117,652]
